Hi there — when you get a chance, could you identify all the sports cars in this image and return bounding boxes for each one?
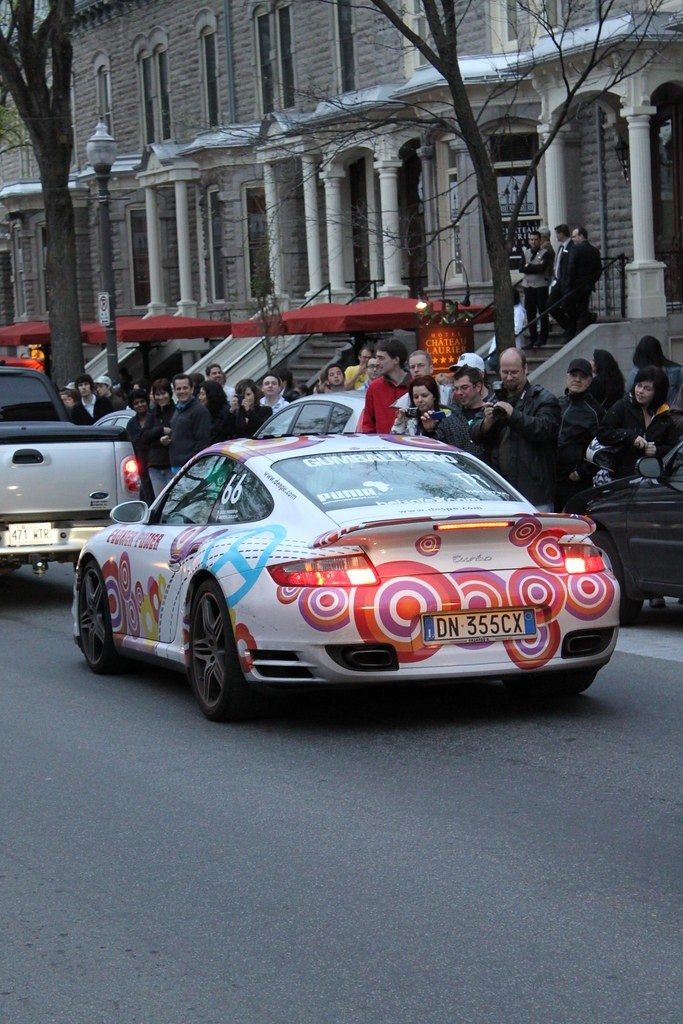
[69,430,623,724]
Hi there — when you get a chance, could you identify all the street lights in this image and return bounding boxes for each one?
[72,122,134,399]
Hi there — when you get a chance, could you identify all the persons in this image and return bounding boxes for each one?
[488,287,532,369]
[538,227,556,286]
[544,224,577,340]
[565,227,603,334]
[589,348,626,413]
[596,365,679,480]
[55,338,563,516]
[625,336,683,409]
[549,358,605,513]
[519,230,551,350]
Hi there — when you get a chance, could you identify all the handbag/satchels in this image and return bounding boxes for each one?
[586,437,617,468]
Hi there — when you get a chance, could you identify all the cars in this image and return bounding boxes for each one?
[250,387,367,440]
[564,440,683,629]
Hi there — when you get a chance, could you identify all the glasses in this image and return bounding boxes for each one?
[453,382,477,391]
[367,365,380,370]
[499,369,523,378]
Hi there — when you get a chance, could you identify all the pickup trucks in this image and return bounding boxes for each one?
[1,359,144,577]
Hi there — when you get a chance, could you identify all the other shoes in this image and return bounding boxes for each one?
[534,341,545,346]
[649,597,666,608]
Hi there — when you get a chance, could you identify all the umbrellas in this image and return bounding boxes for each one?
[1,294,496,395]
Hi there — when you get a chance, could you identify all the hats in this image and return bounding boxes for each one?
[64,382,76,389]
[449,353,485,373]
[94,376,112,387]
[567,358,593,377]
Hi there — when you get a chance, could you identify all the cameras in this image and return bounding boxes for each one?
[406,408,421,418]
[491,399,507,417]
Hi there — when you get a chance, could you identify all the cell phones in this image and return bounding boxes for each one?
[432,411,446,420]
[234,395,244,401]
